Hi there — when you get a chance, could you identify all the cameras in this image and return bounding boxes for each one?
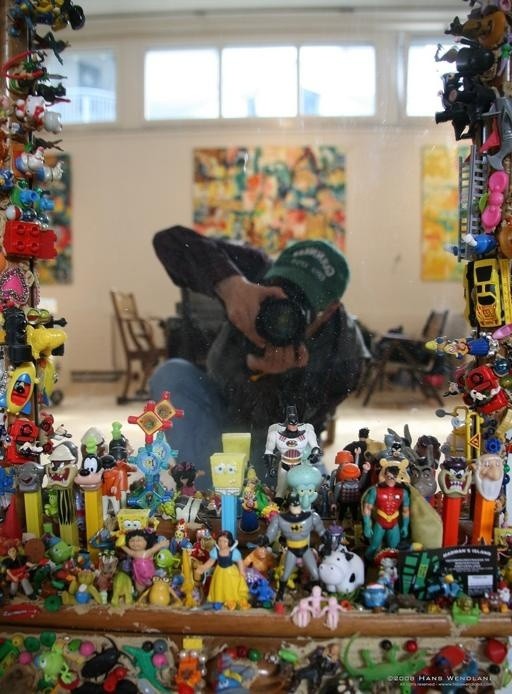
[253,295,316,349]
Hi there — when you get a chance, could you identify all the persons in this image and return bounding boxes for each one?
[0,399,512,693]
[145,224,376,494]
[0,0,94,434]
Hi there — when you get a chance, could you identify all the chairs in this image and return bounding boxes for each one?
[355,308,449,408]
[110,291,168,405]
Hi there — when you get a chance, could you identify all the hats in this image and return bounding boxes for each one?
[264,239,350,316]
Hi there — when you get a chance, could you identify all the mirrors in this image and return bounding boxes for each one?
[0,1,512,638]
